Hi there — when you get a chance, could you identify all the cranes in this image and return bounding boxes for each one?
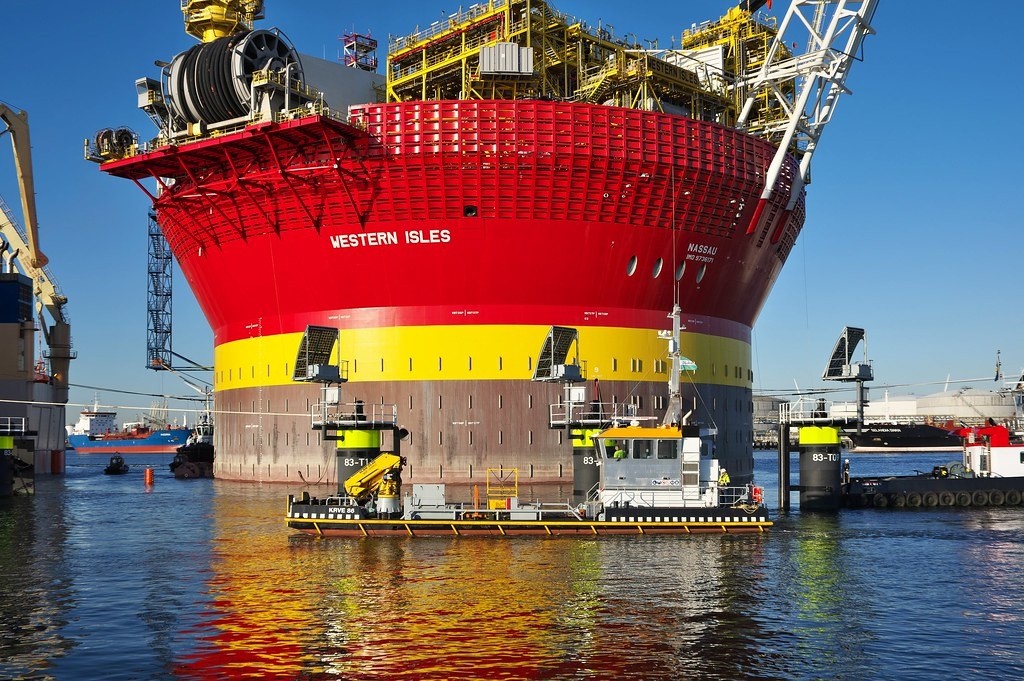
[0,100,78,416]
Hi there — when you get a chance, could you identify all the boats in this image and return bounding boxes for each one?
[841,420,1024,508]
[104,455,129,476]
[284,282,775,539]
[65,397,188,454]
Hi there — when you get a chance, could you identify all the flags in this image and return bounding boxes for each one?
[793,42,798,48]
[995,356,1001,381]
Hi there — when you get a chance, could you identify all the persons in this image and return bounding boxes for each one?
[718,468,730,507]
[614,445,625,459]
[842,459,850,483]
